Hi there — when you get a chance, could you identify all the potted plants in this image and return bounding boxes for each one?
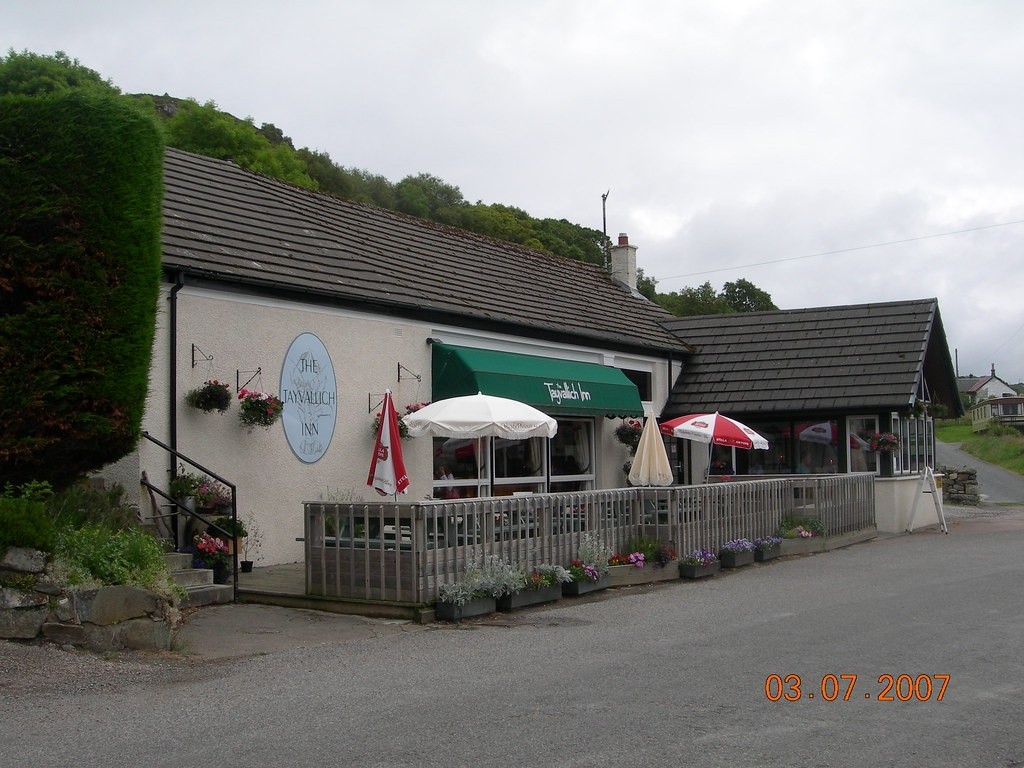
[212,519,248,554]
[170,470,196,516]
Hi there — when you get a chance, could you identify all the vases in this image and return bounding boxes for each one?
[197,496,219,514]
[500,583,563,608]
[757,545,782,562]
[931,411,942,417]
[721,550,754,568]
[678,560,722,577]
[880,453,894,477]
[572,560,677,595]
[436,594,497,618]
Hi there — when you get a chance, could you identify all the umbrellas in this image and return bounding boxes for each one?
[434,432,522,476]
[784,421,867,474]
[401,392,558,497]
[628,411,675,488]
[659,411,769,485]
[367,389,410,501]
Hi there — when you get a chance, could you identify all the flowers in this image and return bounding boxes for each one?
[186,378,232,415]
[621,461,633,474]
[898,399,925,422]
[238,390,285,432]
[756,536,783,549]
[407,400,428,412]
[372,409,410,439]
[869,434,900,452]
[926,404,949,423]
[570,531,674,582]
[684,549,719,563]
[512,566,571,590]
[617,419,644,443]
[721,537,755,553]
[198,479,232,504]
[441,559,525,605]
[192,533,229,568]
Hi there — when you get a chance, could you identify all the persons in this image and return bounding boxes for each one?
[437,462,460,501]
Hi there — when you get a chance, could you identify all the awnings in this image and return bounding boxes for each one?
[431,342,645,420]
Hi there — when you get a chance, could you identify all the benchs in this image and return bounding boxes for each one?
[572,512,648,521]
[520,516,612,528]
[294,537,438,551]
[380,531,482,544]
[645,497,761,515]
[464,520,562,535]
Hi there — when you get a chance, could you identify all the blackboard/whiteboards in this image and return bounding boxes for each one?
[906,466,946,530]
